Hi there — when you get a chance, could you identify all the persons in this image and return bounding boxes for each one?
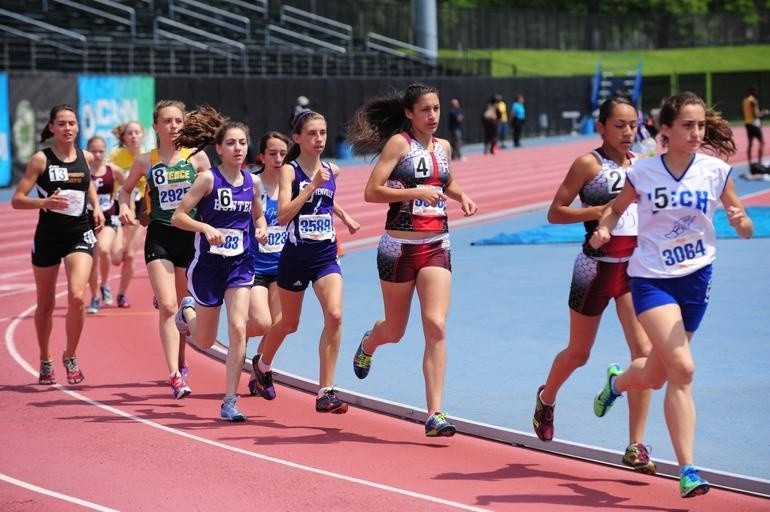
[532,96,656,475]
[742,87,770,175]
[482,93,526,154]
[590,90,754,498]
[447,97,465,160]
[352,81,478,438]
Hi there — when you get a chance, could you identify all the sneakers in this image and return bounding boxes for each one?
[620,439,658,476]
[151,295,160,310]
[247,349,279,401]
[675,462,712,499]
[592,360,626,421]
[99,281,113,306]
[115,292,132,310]
[174,295,196,335]
[219,395,248,422]
[531,382,560,444]
[423,407,458,439]
[350,327,376,380]
[85,293,101,317]
[167,364,191,400]
[314,386,349,416]
[37,351,58,386]
[62,349,84,386]
